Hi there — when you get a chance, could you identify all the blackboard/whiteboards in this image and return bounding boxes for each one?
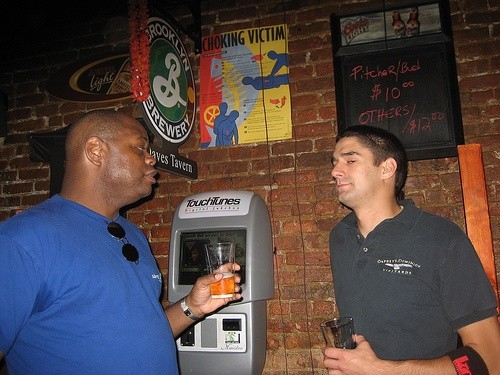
[331,40,465,161]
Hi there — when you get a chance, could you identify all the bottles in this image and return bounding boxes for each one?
[392,10,406,36]
[406,6,420,36]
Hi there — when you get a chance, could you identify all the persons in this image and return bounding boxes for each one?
[0,109,244,375]
[321,123,499,375]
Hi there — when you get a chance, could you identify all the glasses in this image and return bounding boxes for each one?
[106,220,140,265]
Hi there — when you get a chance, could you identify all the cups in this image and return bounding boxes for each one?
[319,316,354,349]
[203,242,235,298]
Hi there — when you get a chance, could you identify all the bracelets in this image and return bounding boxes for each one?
[444,344,489,375]
[180,296,204,322]
